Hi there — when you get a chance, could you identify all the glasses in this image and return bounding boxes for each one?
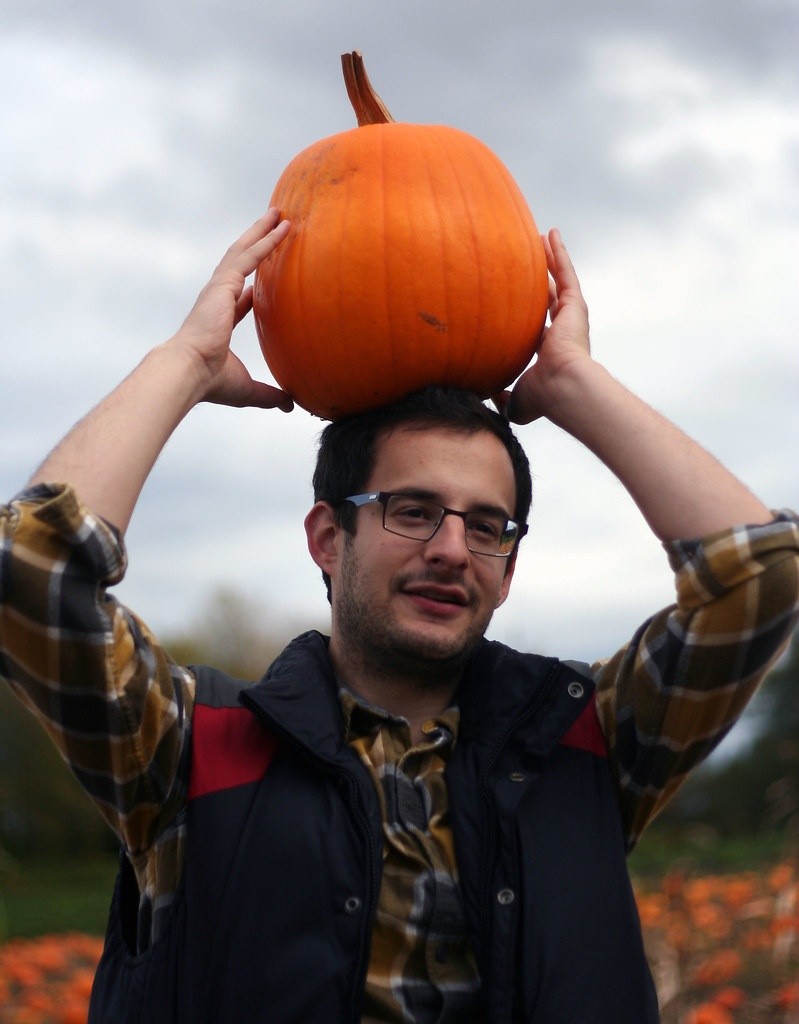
[344,491,529,557]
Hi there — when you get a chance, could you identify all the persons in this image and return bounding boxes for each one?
[0,201,799,1023]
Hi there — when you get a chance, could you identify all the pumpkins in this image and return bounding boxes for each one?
[628,859,799,1024]
[252,50,549,419]
[0,930,108,1024]
